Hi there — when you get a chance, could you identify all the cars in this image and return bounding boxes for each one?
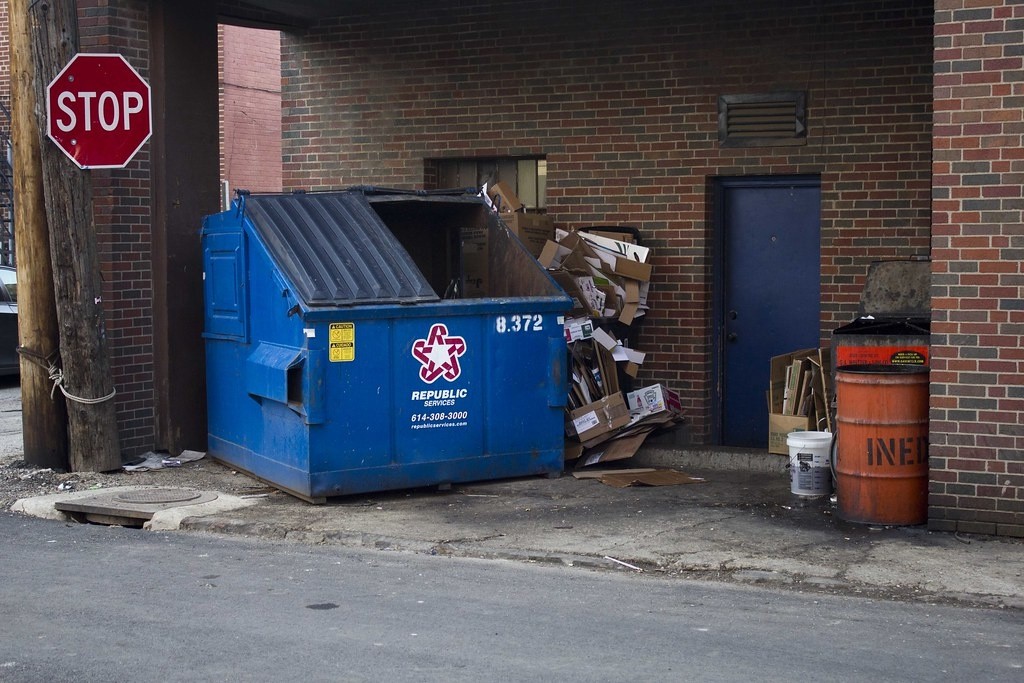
[0,265,21,381]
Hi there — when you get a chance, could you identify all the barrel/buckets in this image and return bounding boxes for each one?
[785,432,833,496]
[835,364,929,525]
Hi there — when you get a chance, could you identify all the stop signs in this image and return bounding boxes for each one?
[44,52,153,170]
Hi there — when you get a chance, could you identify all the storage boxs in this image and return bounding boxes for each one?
[488,181,522,214]
[498,213,554,259]
[627,383,683,422]
[570,391,631,443]
[768,347,818,455]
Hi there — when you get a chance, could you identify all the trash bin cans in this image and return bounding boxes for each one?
[198,183,572,506]
